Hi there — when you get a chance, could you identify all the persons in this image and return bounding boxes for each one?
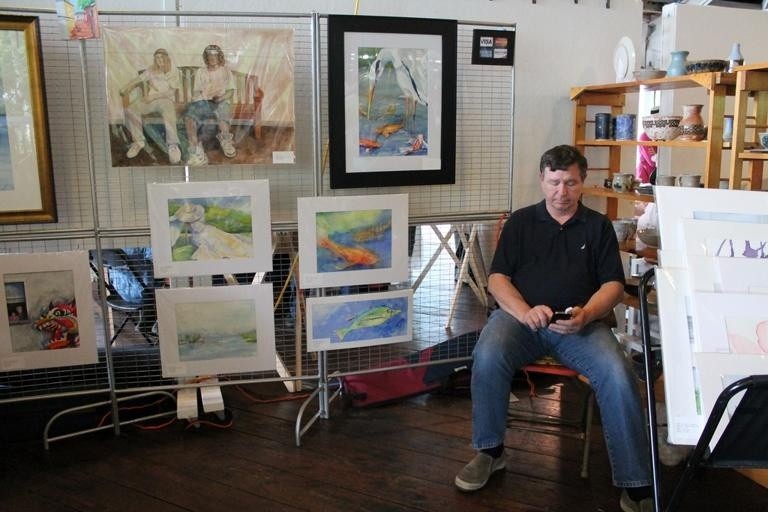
[182,43,236,166]
[119,48,181,165]
[454,143,666,512]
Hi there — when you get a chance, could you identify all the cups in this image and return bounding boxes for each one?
[613,219,629,244]
[611,171,635,192]
[628,217,639,239]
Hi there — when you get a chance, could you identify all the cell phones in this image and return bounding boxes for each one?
[548,313,571,325]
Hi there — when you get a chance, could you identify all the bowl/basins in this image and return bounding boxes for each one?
[641,115,681,141]
[634,229,661,249]
[631,355,663,384]
[633,68,664,81]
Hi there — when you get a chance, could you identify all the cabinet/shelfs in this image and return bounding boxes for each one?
[729,63,768,192]
[569,67,734,374]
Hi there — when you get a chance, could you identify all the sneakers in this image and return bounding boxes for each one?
[455,448,508,493]
[126,141,145,159]
[168,143,182,164]
[619,489,654,512]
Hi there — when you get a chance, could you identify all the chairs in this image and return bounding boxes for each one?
[505,303,618,479]
[89,249,158,350]
[642,375,768,512]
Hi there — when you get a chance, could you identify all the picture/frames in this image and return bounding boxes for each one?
[0,251,99,372]
[1,16,57,226]
[472,28,516,67]
[304,290,411,351]
[154,283,277,379]
[146,180,274,279]
[329,14,457,190]
[298,192,411,290]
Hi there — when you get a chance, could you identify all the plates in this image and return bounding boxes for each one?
[613,36,637,83]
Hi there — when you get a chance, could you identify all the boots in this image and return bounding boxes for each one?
[184,142,209,167]
[216,133,237,158]
